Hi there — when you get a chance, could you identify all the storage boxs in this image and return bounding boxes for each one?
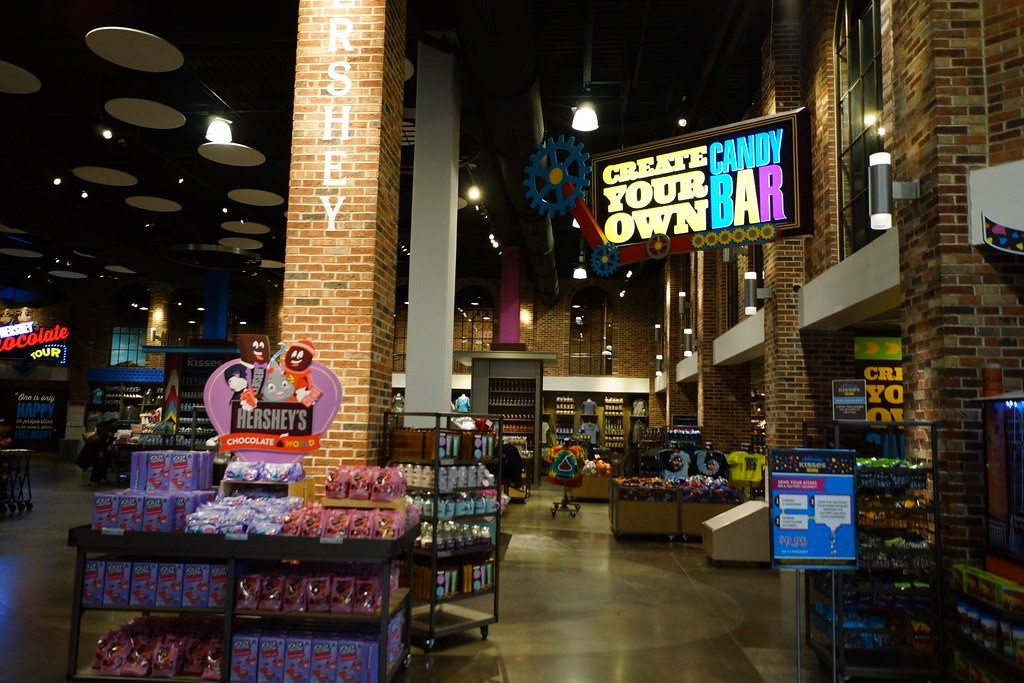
[79,449,403,683]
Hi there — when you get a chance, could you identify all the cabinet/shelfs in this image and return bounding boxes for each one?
[93,383,218,450]
[64,520,423,683]
[552,412,625,453]
[487,377,535,478]
[381,411,503,649]
[801,418,949,682]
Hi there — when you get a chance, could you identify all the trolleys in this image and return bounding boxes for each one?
[381,410,504,651]
[806,415,950,683]
[0,447,33,513]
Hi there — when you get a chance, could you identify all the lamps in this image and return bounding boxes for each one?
[655,354,666,377]
[683,328,698,357]
[679,291,692,314]
[654,324,666,342]
[865,151,919,231]
[744,271,770,315]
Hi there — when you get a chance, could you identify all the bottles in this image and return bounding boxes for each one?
[489,380,535,432]
[166,377,216,446]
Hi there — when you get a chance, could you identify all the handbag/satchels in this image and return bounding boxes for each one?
[83,431,100,445]
[106,441,119,456]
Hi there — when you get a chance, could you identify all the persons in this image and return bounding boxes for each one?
[581,398,597,414]
[76,424,107,483]
[455,393,470,412]
[492,444,523,488]
[392,393,405,409]
[105,418,117,444]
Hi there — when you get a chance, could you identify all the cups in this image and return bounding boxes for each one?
[397,464,499,550]
